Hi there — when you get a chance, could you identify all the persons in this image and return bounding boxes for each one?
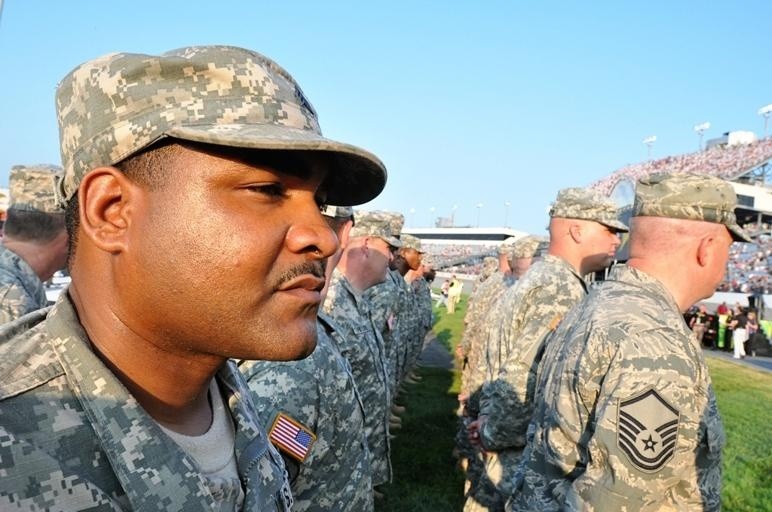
[0,165,68,326]
[1,46,388,509]
[458,173,752,511]
[234,210,436,509]
[419,244,498,274]
[431,276,463,314]
[589,132,771,359]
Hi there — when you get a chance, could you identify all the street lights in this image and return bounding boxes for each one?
[757,104,772,138]
[451,205,457,228]
[409,209,417,228]
[504,202,510,228]
[643,134,658,161]
[476,203,483,228]
[428,207,436,227]
[695,121,709,155]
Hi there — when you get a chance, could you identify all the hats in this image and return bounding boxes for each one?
[53,41,389,207]
[7,162,69,216]
[400,234,438,273]
[321,202,357,227]
[631,170,757,245]
[548,186,632,233]
[482,234,551,267]
[349,208,404,248]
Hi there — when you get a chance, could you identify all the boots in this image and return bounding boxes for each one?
[391,403,406,414]
[405,359,425,385]
[394,387,409,399]
[389,412,402,434]
